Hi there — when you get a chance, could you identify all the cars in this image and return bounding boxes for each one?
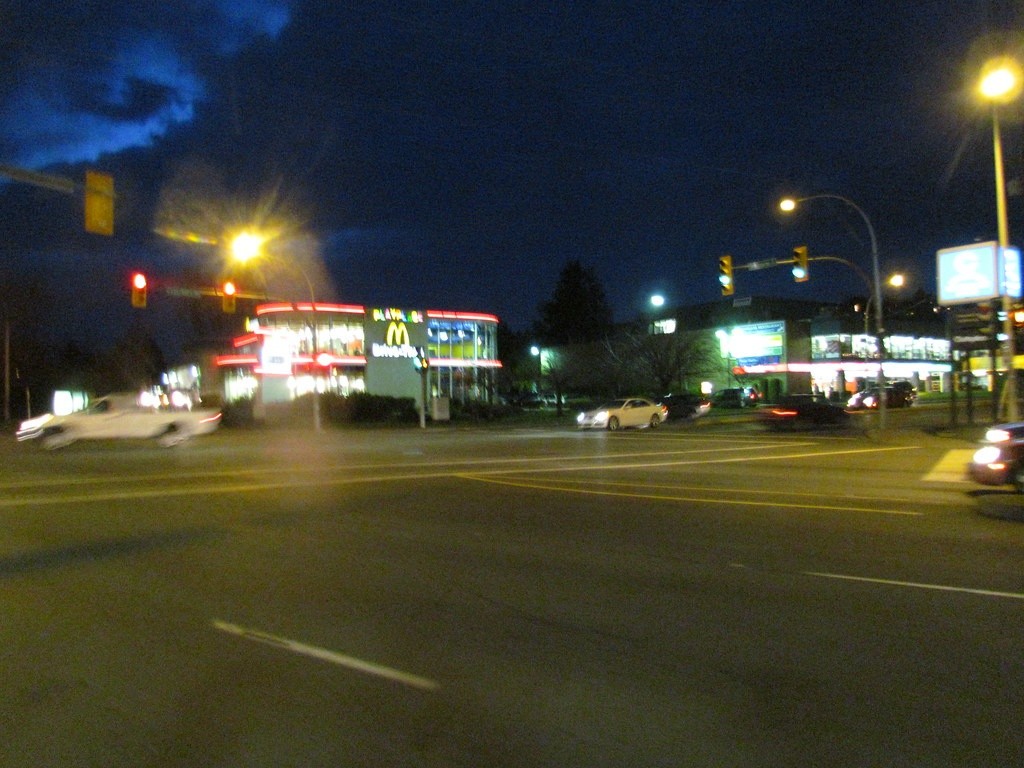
[708,388,758,408]
[576,397,669,432]
[850,380,916,409]
[658,392,711,420]
[14,391,224,450]
[500,390,566,409]
[966,420,1024,494]
[760,394,848,430]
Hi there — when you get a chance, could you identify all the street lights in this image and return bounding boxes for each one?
[983,62,1015,423]
[865,275,905,395]
[230,233,326,435]
[780,195,888,429]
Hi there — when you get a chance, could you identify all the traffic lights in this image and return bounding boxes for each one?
[224,281,237,313]
[718,253,735,298]
[132,273,146,307]
[791,245,808,285]
